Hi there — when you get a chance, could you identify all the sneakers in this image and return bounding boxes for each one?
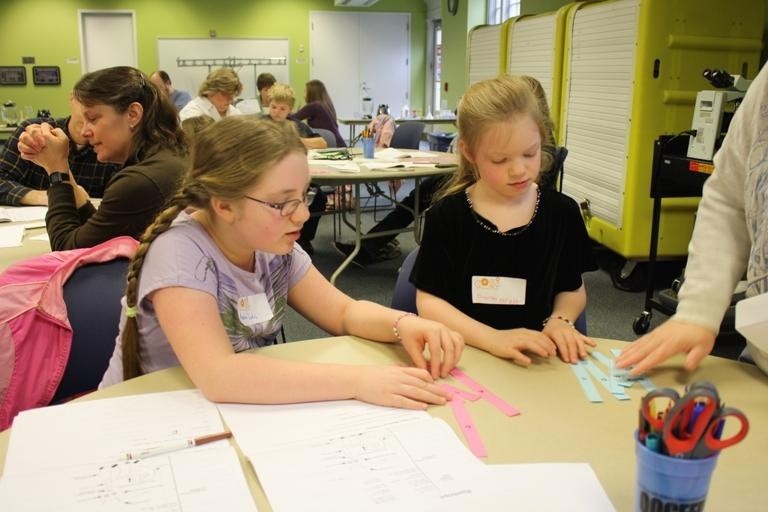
[331,240,367,269]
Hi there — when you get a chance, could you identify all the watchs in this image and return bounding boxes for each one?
[48,171,70,185]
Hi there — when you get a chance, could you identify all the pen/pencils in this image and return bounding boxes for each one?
[126,431,232,462]
[639,397,726,451]
[362,124,376,139]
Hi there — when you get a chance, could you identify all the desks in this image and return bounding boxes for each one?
[0,124,18,140]
[339,118,459,148]
[0,335,768,512]
[306,148,463,286]
[0,198,102,278]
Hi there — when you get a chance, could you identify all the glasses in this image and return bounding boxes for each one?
[243,186,318,218]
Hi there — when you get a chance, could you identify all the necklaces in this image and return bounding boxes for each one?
[464,180,542,237]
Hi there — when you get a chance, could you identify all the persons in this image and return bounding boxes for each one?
[260,83,327,255]
[406,74,597,367]
[330,76,556,269]
[149,67,348,148]
[17,66,191,252]
[0,87,120,205]
[616,57,767,381]
[93,113,467,412]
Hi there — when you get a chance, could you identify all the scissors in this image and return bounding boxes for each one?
[641,381,748,459]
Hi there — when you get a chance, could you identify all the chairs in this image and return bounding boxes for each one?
[539,147,567,194]
[393,247,586,336]
[311,128,337,148]
[0,239,136,429]
[359,122,425,223]
[709,332,747,359]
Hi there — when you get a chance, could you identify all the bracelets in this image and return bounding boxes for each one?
[392,312,417,342]
[541,315,577,330]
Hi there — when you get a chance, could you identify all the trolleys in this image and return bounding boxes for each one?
[625,132,746,358]
